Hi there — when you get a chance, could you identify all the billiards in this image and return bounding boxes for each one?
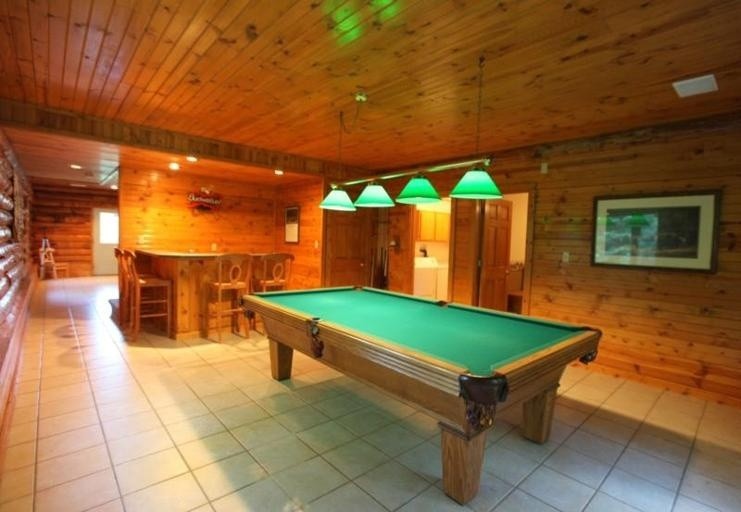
[585,352,596,360]
[467,405,493,430]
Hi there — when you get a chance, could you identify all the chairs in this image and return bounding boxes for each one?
[113,247,174,342]
[199,252,294,340]
[36,238,70,280]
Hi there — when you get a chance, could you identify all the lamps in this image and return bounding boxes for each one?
[320,156,504,214]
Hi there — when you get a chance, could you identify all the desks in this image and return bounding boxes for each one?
[239,281,601,507]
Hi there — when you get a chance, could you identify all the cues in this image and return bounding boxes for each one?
[370,247,388,289]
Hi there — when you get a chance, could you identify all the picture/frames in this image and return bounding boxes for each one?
[592,188,722,275]
